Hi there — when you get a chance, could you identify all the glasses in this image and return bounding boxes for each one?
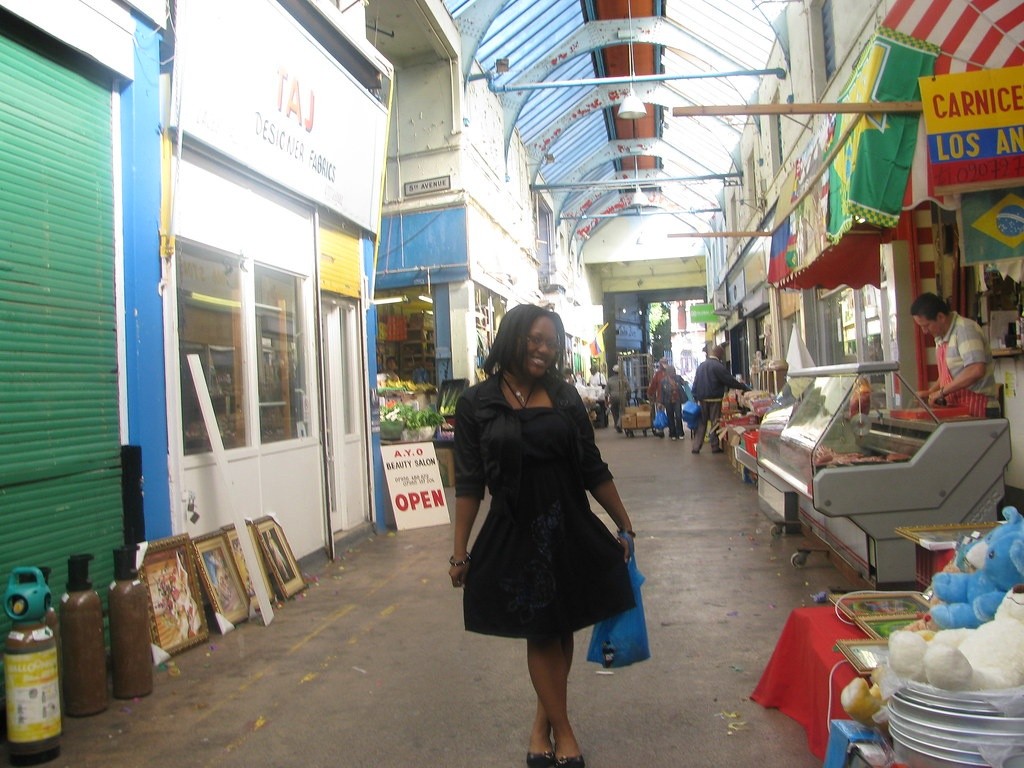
[525,334,560,351]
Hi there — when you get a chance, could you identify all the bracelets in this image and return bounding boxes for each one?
[449,553,470,566]
[617,528,636,537]
[939,387,948,397]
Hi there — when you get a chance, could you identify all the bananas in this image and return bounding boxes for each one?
[385,377,435,390]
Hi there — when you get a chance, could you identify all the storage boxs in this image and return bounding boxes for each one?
[730,426,759,447]
[732,447,738,469]
[744,432,758,457]
[436,378,470,418]
[624,404,653,414]
[710,423,737,462]
[435,448,455,488]
[914,542,956,587]
[621,413,637,428]
[636,411,652,428]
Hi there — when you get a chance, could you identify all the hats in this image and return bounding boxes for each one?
[612,364,620,371]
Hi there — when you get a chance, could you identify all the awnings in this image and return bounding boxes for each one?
[765,0,1024,291]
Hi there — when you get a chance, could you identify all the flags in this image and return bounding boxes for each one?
[785,322,816,398]
[589,339,602,356]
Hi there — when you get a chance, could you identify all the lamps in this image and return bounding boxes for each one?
[615,0,647,121]
[630,185,652,207]
[496,58,510,73]
[525,153,555,167]
[739,199,761,213]
[374,294,408,305]
[580,210,587,219]
[634,229,651,245]
[419,293,433,304]
[581,232,590,240]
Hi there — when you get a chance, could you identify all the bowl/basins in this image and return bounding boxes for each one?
[887,679,1024,768]
[402,426,436,442]
[434,440,454,449]
[381,421,403,439]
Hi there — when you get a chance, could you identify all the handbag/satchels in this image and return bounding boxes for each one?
[586,532,651,669]
[599,373,607,389]
[653,408,668,430]
[682,400,702,430]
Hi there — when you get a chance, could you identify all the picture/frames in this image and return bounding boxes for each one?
[136,533,209,666]
[251,511,308,599]
[893,520,1006,550]
[835,636,890,675]
[220,518,278,613]
[826,591,932,621]
[852,611,928,640]
[191,530,253,634]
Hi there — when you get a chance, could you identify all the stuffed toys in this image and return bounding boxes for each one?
[840,506,1024,736]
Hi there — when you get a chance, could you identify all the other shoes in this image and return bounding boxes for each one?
[712,448,724,454]
[554,738,586,768]
[527,739,555,768]
[692,450,700,454]
[672,437,676,440]
[679,436,684,439]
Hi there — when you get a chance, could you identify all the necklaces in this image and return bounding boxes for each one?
[501,374,535,408]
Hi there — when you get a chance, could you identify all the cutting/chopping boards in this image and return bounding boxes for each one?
[890,406,969,419]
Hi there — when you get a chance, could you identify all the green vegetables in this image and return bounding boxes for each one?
[380,403,447,431]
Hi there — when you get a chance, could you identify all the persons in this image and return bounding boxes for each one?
[910,293,1001,419]
[266,530,289,578]
[449,305,636,768]
[587,365,609,426]
[604,365,631,433]
[660,365,689,440]
[648,359,671,438]
[692,346,752,454]
[380,357,399,381]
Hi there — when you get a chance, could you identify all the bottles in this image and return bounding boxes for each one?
[403,392,419,411]
